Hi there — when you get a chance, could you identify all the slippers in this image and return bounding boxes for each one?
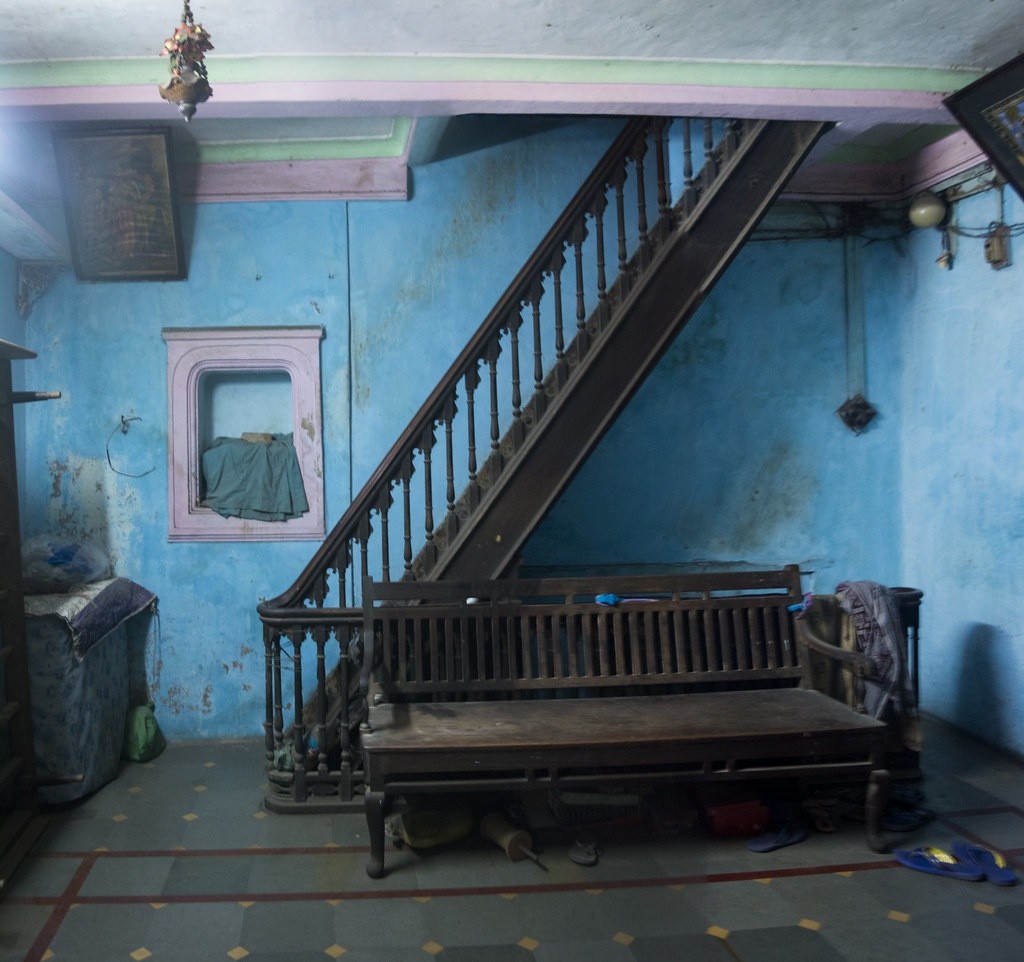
[745,823,804,852]
[950,841,1019,886]
[815,816,835,832]
[881,806,935,831]
[569,831,599,865]
[894,845,981,881]
[887,789,925,805]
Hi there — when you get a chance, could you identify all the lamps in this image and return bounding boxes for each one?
[158,0,214,123]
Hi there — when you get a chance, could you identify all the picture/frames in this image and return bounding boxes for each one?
[50,119,183,282]
[941,55,1024,202]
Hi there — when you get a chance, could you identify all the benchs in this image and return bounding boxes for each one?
[361,576,895,878]
